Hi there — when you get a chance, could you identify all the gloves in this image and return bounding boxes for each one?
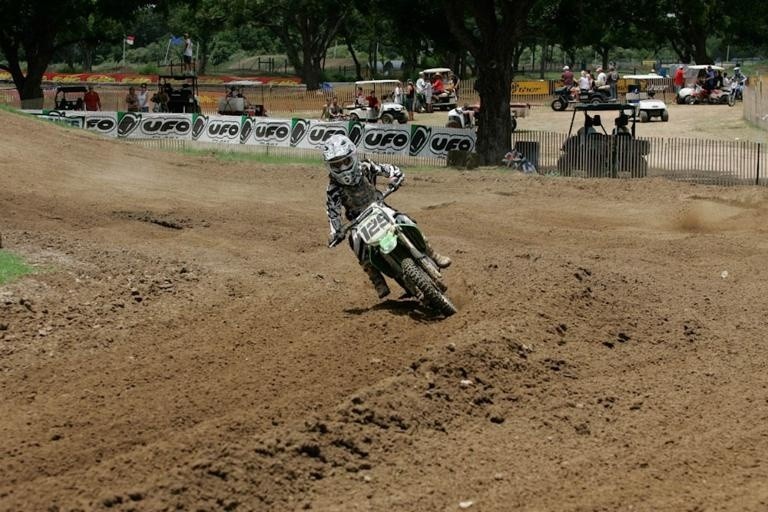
[388,176,402,191]
[329,231,345,247]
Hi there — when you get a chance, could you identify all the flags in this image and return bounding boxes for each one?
[126,36,136,46]
[171,37,181,45]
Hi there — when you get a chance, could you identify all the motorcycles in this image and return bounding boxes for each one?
[326,185,459,318]
[727,79,742,107]
[550,85,610,111]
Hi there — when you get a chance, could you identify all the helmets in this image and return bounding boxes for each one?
[323,134,357,185]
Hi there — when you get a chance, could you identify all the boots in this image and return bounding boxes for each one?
[369,271,390,298]
[429,248,452,268]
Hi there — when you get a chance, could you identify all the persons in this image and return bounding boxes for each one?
[329,97,344,122]
[83,84,102,111]
[393,82,404,105]
[643,80,655,98]
[137,83,150,112]
[182,33,194,77]
[403,79,414,122]
[413,71,460,113]
[355,87,364,105]
[366,91,382,112]
[321,133,453,296]
[126,87,141,112]
[562,64,621,102]
[227,86,237,98]
[697,67,748,95]
[670,66,685,104]
